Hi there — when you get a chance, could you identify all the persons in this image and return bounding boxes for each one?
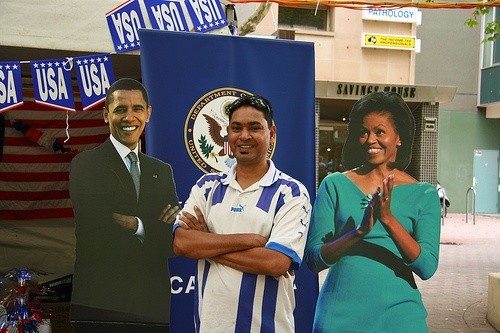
[304,91,441,333]
[172,95,312,333]
[69,79,181,333]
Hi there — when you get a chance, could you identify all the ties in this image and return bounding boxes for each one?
[128,152,139,203]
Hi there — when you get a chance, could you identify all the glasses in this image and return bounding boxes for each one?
[226,96,271,113]
